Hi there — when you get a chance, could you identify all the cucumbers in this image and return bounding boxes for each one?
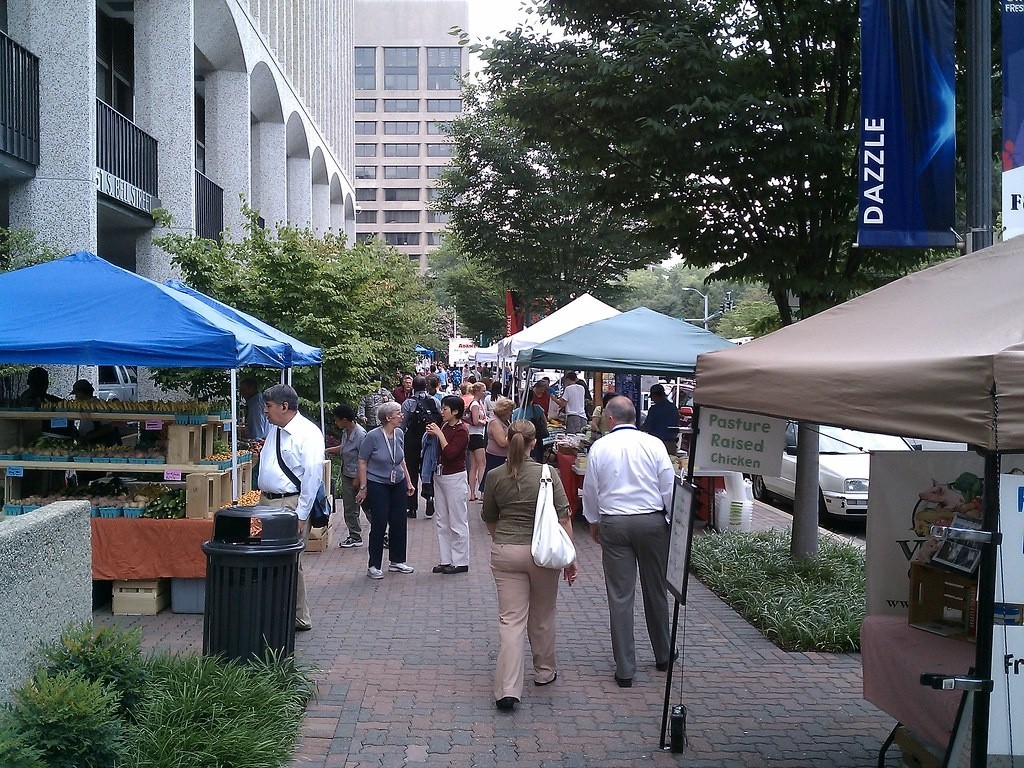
[140,488,188,519]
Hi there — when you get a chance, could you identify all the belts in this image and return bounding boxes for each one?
[263,492,301,500]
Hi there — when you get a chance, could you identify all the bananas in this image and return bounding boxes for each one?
[27,436,134,450]
[40,399,228,417]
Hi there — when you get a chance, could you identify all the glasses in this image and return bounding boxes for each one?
[391,412,404,419]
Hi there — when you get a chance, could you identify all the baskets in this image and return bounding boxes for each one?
[0,407,252,518]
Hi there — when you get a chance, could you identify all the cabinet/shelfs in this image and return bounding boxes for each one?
[0,411,252,519]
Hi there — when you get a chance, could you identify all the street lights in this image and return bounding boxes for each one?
[682,287,710,332]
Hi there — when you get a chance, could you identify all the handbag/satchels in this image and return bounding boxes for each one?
[309,481,331,528]
[530,463,576,568]
[547,395,560,422]
[461,400,480,426]
[531,405,549,439]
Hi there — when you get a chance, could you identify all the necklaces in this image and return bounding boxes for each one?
[610,426,638,433]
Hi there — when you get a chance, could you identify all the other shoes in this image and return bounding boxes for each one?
[533,670,557,686]
[407,509,416,518]
[425,499,434,516]
[496,696,517,709]
[295,617,312,630]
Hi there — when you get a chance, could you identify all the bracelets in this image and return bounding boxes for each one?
[384,397,390,402]
[360,485,367,490]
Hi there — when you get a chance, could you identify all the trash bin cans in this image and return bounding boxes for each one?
[201,505,307,665]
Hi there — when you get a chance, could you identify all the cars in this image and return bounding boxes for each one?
[751,417,923,521]
[665,384,695,453]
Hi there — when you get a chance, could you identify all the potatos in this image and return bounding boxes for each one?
[9,493,150,509]
[0,446,167,458]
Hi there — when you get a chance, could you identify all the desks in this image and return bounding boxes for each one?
[541,439,735,533]
[856,611,1024,768]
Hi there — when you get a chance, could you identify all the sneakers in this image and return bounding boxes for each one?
[443,564,468,574]
[433,564,450,573]
[367,566,384,579]
[338,536,363,547]
[383,534,389,548]
[388,561,414,573]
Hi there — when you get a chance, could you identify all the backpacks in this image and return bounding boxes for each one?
[409,391,443,440]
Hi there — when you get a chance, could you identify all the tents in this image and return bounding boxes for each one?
[0,250,324,505]
[659,234,1024,767]
[414,344,434,363]
[467,292,739,422]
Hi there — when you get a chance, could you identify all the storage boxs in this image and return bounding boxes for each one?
[111,577,161,616]
[908,560,978,642]
[0,403,231,424]
[305,459,333,553]
[712,491,753,537]
[0,448,165,464]
[894,725,942,768]
[198,444,253,470]
[171,576,206,614]
[4,498,145,519]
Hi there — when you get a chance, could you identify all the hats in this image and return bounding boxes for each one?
[371,375,382,382]
[70,379,94,392]
[648,385,665,397]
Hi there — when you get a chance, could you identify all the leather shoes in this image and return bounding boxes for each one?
[656,646,679,671]
[614,672,633,688]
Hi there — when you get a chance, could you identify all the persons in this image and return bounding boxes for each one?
[326,359,678,581]
[255,383,325,630]
[240,377,274,439]
[68,379,113,438]
[481,419,579,709]
[19,368,79,437]
[582,395,679,687]
